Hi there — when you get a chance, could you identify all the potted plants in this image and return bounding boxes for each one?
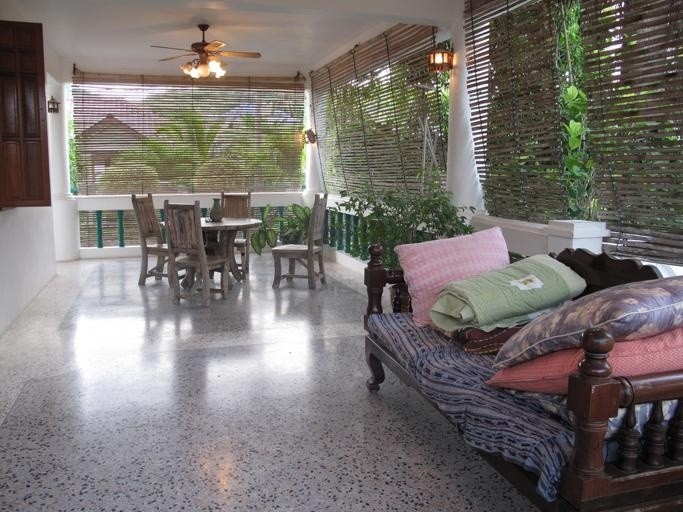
[329,169,479,312]
[246,201,315,255]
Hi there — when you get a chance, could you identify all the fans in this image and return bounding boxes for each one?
[149,24,261,67]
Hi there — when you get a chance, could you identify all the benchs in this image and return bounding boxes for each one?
[361,235,682,512]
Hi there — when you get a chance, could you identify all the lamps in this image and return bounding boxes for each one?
[180,53,226,79]
[427,41,455,72]
[48,96,61,114]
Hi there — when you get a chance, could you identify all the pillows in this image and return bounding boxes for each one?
[505,387,680,437]
[491,274,681,369]
[484,329,682,397]
[394,226,509,332]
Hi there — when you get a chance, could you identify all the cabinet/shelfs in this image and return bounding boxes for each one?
[0,19,52,212]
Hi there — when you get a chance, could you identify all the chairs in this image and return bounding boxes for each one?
[131,193,190,287]
[219,190,251,273]
[163,200,231,307]
[270,193,328,291]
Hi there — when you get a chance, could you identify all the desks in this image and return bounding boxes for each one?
[158,217,263,287]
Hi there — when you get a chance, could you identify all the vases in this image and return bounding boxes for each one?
[209,198,224,222]
